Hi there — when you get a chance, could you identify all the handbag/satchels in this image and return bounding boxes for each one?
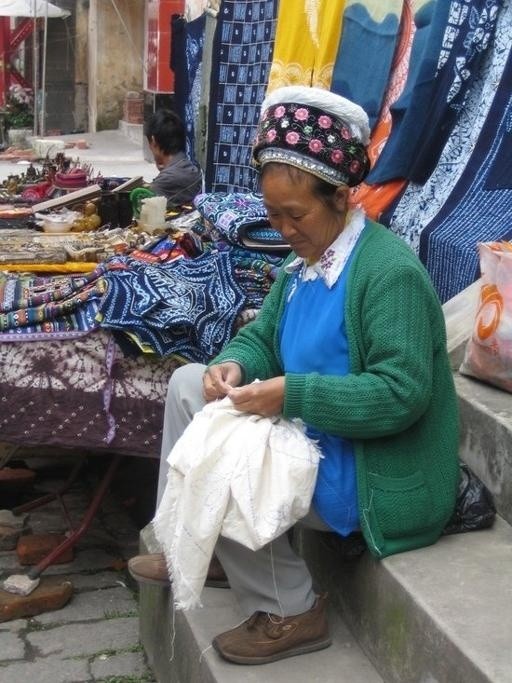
[459,240,512,393]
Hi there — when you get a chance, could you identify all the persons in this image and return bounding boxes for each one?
[125,138,459,666]
[141,104,208,220]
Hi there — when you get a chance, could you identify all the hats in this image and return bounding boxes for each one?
[252,86,371,187]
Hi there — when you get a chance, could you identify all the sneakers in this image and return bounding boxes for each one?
[127,553,230,587]
[212,595,332,664]
